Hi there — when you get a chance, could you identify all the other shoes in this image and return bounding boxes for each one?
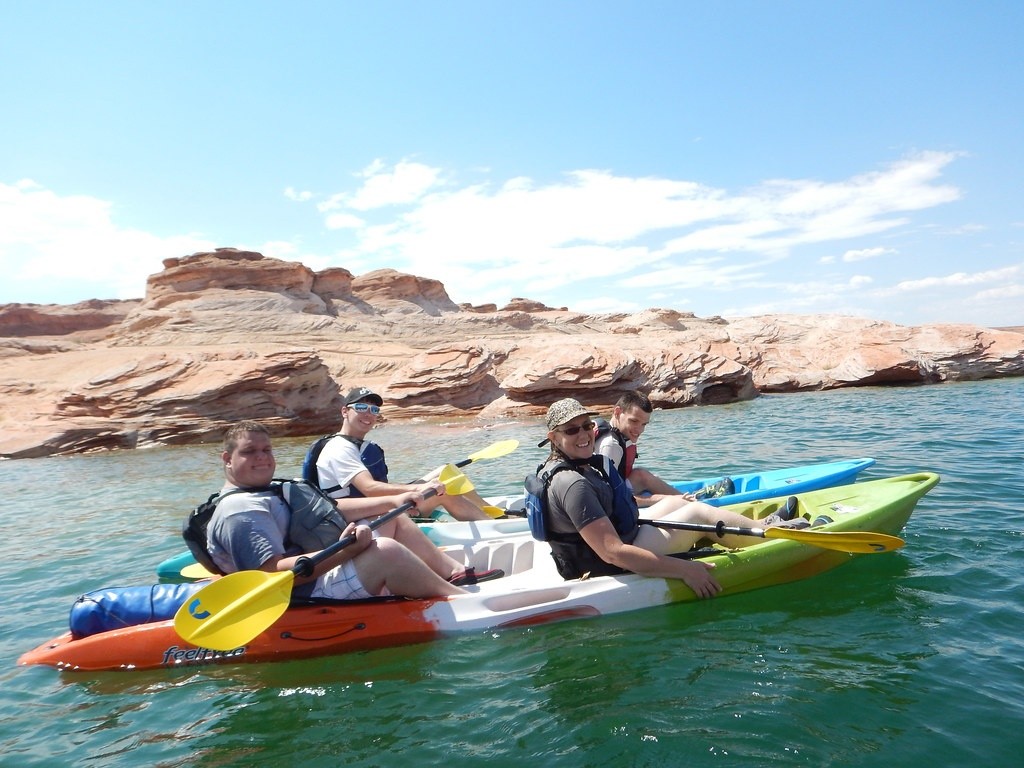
[812,515,834,527]
[446,566,505,586]
[759,496,798,529]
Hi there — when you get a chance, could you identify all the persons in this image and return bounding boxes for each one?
[302,386,527,521]
[205,421,505,606]
[524,390,834,600]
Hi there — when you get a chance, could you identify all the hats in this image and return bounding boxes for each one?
[345,386,383,407]
[538,397,599,448]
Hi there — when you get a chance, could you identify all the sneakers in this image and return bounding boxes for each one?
[692,477,735,499]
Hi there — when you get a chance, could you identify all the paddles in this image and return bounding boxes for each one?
[172,463,476,651]
[481,504,906,554]
[179,439,520,580]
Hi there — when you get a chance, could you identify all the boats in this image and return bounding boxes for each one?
[12,471,938,675]
[159,459,869,582]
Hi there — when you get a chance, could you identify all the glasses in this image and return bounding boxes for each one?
[556,422,595,435]
[347,403,380,415]
